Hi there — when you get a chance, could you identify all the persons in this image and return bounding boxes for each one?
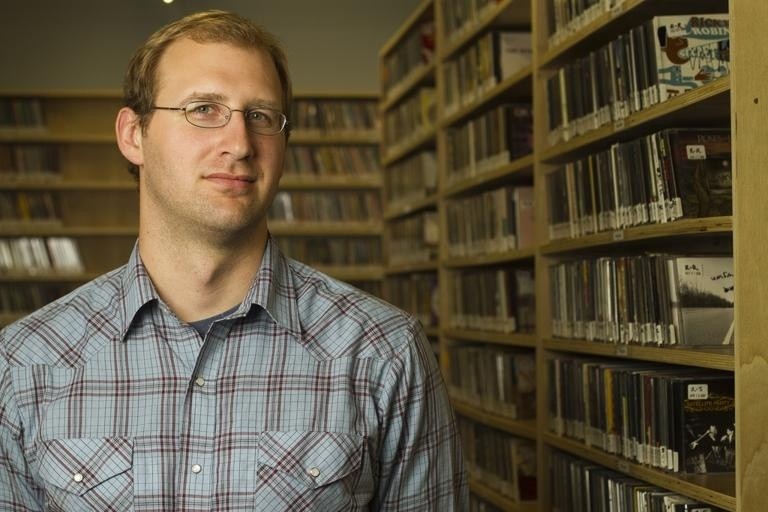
[718,425,737,472]
[689,423,726,473]
[0,11,471,512]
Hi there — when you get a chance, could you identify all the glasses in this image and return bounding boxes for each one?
[133,101,289,136]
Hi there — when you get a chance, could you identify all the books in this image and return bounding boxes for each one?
[545,1,737,512]
[438,1,538,500]
[379,2,438,357]
[270,99,379,295]
[2,99,89,324]
